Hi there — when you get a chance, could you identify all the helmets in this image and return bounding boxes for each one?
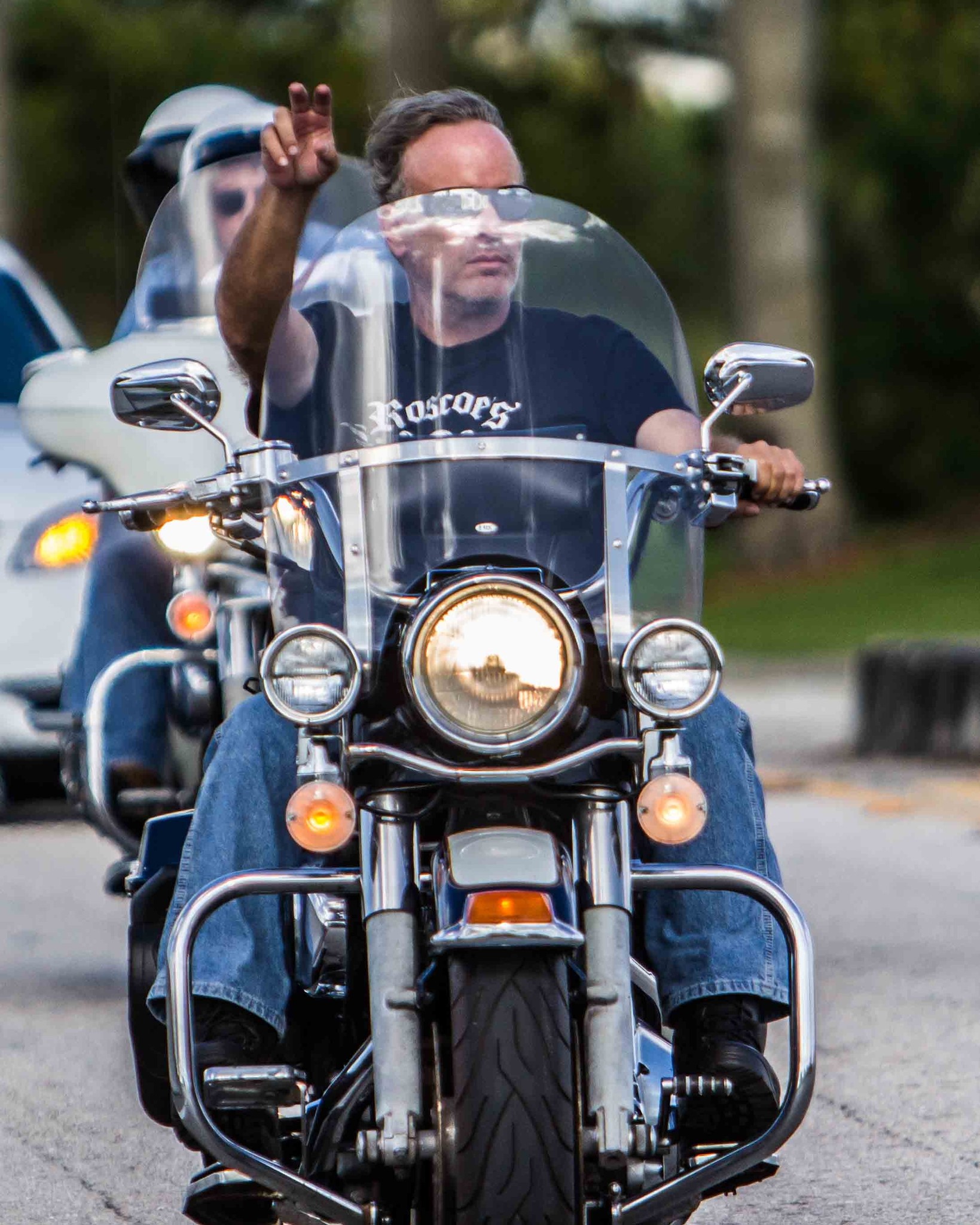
[179,98,277,201]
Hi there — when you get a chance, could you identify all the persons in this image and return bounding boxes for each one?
[63,151,414,895]
[107,79,806,1224]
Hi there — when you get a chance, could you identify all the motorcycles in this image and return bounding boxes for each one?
[16,147,415,984]
[83,184,834,1223]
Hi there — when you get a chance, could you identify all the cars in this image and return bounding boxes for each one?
[0,235,110,753]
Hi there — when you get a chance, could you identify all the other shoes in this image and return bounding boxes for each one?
[106,809,160,898]
[195,1026,284,1187]
[669,989,782,1169]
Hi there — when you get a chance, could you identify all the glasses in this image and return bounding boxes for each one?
[401,185,533,221]
[211,189,262,217]
